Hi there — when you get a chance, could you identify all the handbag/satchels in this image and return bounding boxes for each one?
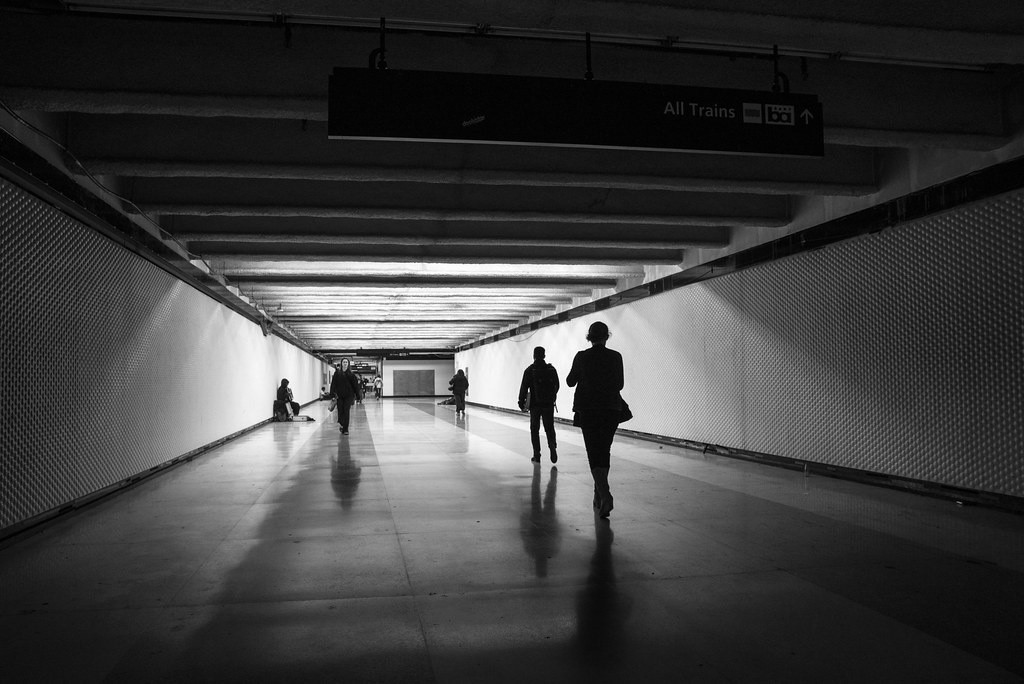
[328,398,337,411]
[619,395,633,425]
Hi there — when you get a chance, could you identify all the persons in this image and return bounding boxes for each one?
[518,347,560,463]
[567,321,632,516]
[449,369,469,416]
[276,378,300,416]
[320,375,383,404]
[330,359,361,435]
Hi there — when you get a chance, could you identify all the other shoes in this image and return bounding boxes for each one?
[339,425,343,432]
[550,444,557,463]
[531,456,540,463]
[598,493,613,518]
[345,429,349,435]
[593,491,601,509]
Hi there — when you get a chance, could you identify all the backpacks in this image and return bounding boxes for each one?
[530,363,556,403]
